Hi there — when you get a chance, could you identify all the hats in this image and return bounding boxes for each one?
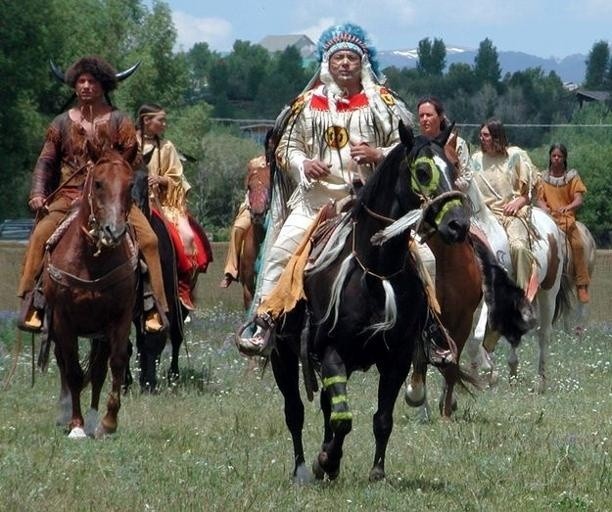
[315,24,387,85]
[67,55,119,93]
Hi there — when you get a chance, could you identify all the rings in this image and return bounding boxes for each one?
[354,156,360,162]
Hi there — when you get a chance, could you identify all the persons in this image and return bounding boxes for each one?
[470,117,537,323]
[412,95,515,282]
[16,55,171,334]
[240,23,437,350]
[220,128,277,289]
[135,103,195,310]
[533,142,591,302]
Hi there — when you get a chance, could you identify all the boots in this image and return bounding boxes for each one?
[176,273,197,312]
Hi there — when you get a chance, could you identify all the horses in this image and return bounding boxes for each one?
[238,119,597,487]
[42,135,214,440]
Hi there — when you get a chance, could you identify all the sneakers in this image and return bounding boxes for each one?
[423,335,451,364]
[577,286,590,303]
[520,301,535,328]
[220,274,232,288]
[24,309,43,329]
[144,313,161,332]
[237,335,264,352]
